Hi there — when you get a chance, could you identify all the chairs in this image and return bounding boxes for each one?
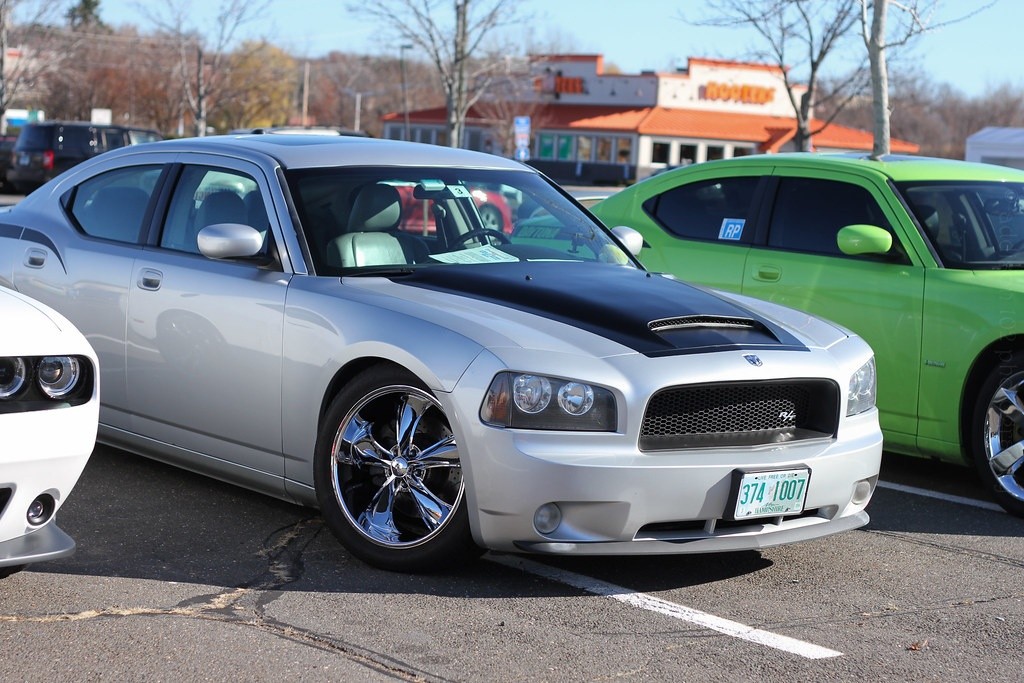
[330,185,489,275]
[194,188,271,263]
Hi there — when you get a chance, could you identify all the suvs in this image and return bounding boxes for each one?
[0,117,178,196]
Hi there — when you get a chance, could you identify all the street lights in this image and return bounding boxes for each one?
[343,90,362,134]
[400,44,415,142]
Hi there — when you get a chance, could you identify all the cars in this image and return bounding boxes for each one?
[499,151,1024,517]
[383,182,513,244]
[0,130,884,570]
[194,128,375,212]
[0,288,101,571]
[494,182,523,212]
